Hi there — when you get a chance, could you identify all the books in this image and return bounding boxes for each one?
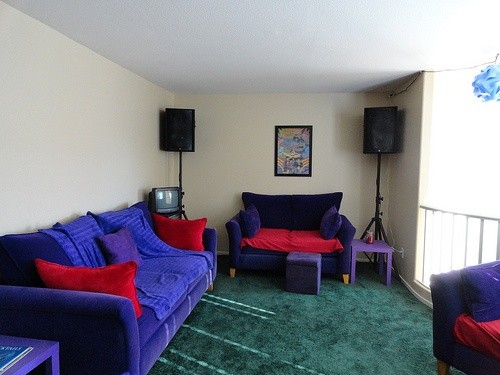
[0,345,34,375]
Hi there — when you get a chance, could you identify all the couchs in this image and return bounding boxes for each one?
[0,201,217,375]
[225,191,357,285]
[430,259,500,375]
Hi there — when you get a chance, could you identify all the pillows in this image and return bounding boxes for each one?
[240,205,261,238]
[460,260,500,322]
[35,258,143,318]
[152,213,207,252]
[95,227,142,265]
[320,206,342,240]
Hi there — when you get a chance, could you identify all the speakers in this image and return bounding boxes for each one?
[363,106,398,154]
[164,108,195,152]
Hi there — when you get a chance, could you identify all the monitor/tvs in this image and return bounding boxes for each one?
[148,186,181,215]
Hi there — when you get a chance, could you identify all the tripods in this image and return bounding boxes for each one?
[360,154,401,281]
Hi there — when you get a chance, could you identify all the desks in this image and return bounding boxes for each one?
[0,335,60,375]
[351,239,392,287]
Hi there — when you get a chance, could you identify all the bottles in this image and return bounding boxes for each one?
[367,232,374,243]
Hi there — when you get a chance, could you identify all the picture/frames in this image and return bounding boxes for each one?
[275,125,312,177]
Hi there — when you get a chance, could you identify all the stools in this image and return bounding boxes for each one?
[286,252,322,294]
